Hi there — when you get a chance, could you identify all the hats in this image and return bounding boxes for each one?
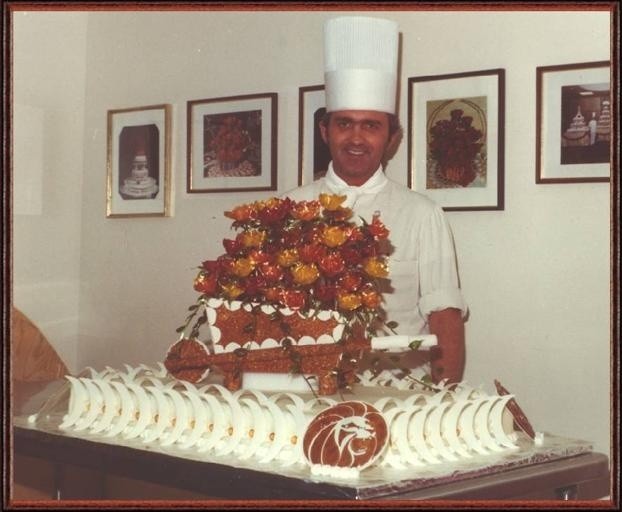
[323,16,399,114]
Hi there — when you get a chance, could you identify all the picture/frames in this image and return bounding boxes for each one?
[186,92,280,192]
[406,69,505,212]
[534,59,614,185]
[296,84,386,188]
[106,103,172,216]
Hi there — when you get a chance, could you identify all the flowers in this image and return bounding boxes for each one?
[173,182,448,403]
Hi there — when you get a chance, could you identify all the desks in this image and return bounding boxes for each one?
[9,415,608,502]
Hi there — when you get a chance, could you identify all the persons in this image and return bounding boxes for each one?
[588,117,597,145]
[280,109,468,390]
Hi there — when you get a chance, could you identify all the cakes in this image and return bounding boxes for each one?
[63,193,514,473]
[123,151,155,197]
[566,107,587,149]
[598,101,612,141]
